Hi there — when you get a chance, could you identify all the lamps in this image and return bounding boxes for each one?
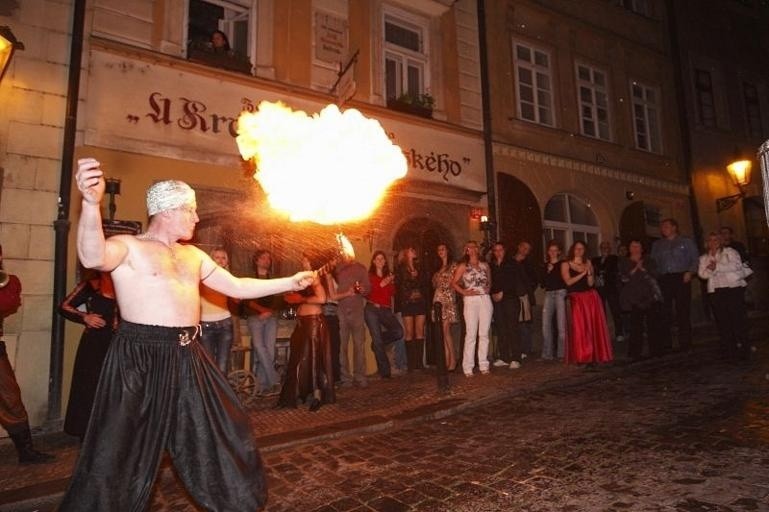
[715,157,754,215]
[478,213,495,259]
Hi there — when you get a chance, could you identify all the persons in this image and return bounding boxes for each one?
[56,157,314,512]
[209,31,231,54]
[0,245,57,465]
[56,268,122,438]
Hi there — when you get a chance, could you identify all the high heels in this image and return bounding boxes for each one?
[447,362,458,372]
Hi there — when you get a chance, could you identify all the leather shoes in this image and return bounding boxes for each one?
[309,398,322,412]
[272,398,295,410]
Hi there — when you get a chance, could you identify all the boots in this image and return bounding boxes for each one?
[6,423,57,463]
[404,337,426,371]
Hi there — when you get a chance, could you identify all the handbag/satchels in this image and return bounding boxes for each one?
[724,263,755,284]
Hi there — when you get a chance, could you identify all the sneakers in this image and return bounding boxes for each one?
[382,375,389,379]
[261,391,272,397]
[371,341,376,352]
[481,370,489,375]
[509,360,521,369]
[272,382,283,395]
[465,372,473,377]
[493,359,509,367]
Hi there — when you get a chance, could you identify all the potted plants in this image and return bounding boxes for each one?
[385,89,435,122]
